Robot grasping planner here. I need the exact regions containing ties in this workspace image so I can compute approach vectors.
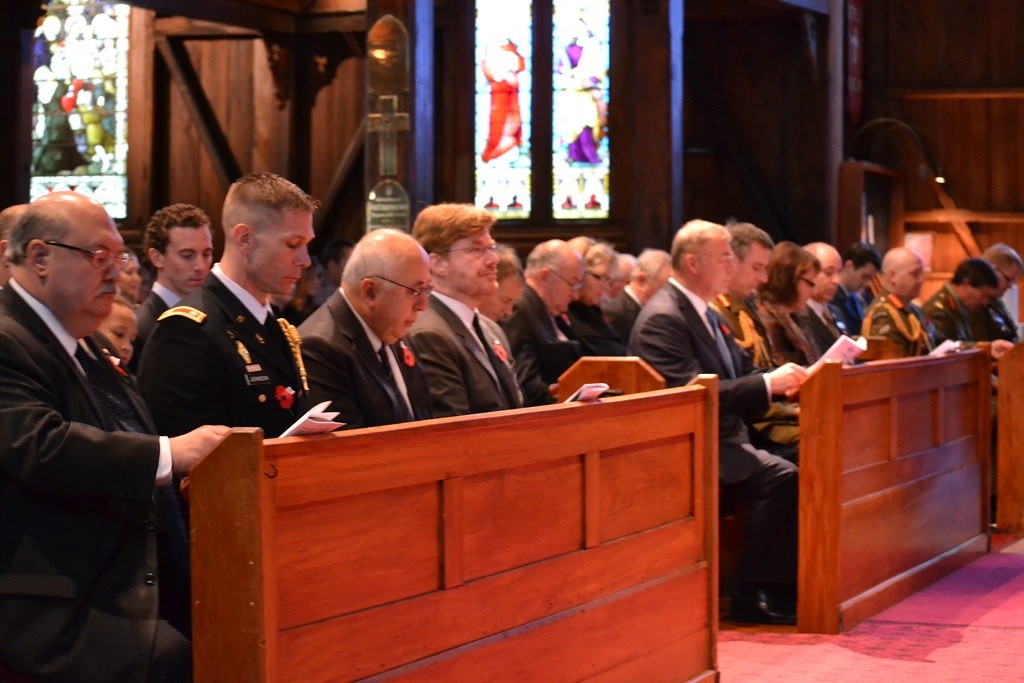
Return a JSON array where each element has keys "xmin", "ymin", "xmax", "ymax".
[
  {"xmin": 706, "ymin": 307, "xmax": 736, "ymax": 378},
  {"xmin": 378, "ymin": 342, "xmax": 413, "ymax": 422},
  {"xmin": 473, "ymin": 314, "xmax": 523, "ymax": 408},
  {"xmin": 824, "ymin": 314, "xmax": 840, "ymax": 338},
  {"xmin": 73, "ymin": 341, "xmax": 190, "ymax": 563},
  {"xmin": 264, "ymin": 311, "xmax": 300, "ymax": 377}
]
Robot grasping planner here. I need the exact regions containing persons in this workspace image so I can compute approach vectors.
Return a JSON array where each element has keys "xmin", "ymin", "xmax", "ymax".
[
  {"xmin": 802, "ymin": 242, "xmax": 864, "ymax": 366},
  {"xmin": 97, "ymin": 203, "xmax": 213, "ymax": 365},
  {"xmin": 709, "ymin": 222, "xmax": 800, "ymax": 464},
  {"xmin": 861, "ymin": 246, "xmax": 1015, "ymax": 420},
  {"xmin": 0, "ymin": 191, "xmax": 230, "ymax": 683},
  {"xmin": 755, "ymin": 241, "xmax": 821, "ymax": 365},
  {"xmin": 138, "ymin": 171, "xmax": 322, "ymax": 638},
  {"xmin": 628, "ymin": 218, "xmax": 808, "ymax": 624},
  {"xmin": 0, "ymin": 204, "xmax": 30, "ymax": 289},
  {"xmin": 827, "ymin": 241, "xmax": 885, "ymax": 334},
  {"xmin": 405, "ymin": 203, "xmax": 526, "ymax": 422},
  {"xmin": 922, "ymin": 258, "xmax": 999, "ymax": 340},
  {"xmin": 496, "ymin": 237, "xmax": 673, "ymax": 408},
  {"xmin": 272, "ymin": 236, "xmax": 357, "ymax": 327},
  {"xmin": 981, "ymin": 243, "xmax": 1024, "ymax": 337},
  {"xmin": 299, "ymin": 227, "xmax": 434, "ymax": 429}
]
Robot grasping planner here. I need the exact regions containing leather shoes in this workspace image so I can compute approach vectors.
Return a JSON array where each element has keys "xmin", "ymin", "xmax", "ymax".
[{"xmin": 729, "ymin": 589, "xmax": 796, "ymax": 623}]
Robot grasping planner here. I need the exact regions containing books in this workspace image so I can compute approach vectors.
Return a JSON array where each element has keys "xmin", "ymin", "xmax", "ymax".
[
  {"xmin": 807, "ymin": 335, "xmax": 867, "ymax": 369},
  {"xmin": 279, "ymin": 401, "xmax": 346, "ymax": 438},
  {"xmin": 564, "ymin": 383, "xmax": 609, "ymax": 401}
]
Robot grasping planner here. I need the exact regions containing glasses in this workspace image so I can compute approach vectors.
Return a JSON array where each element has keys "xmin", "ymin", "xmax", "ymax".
[
  {"xmin": 997, "ymin": 267, "xmax": 1015, "ymax": 286},
  {"xmin": 22, "ymin": 238, "xmax": 133, "ymax": 270},
  {"xmin": 361, "ymin": 275, "xmax": 435, "ymax": 300},
  {"xmin": 798, "ymin": 276, "xmax": 817, "ymax": 292},
  {"xmin": 549, "ymin": 268, "xmax": 583, "ymax": 291},
  {"xmin": 441, "ymin": 244, "xmax": 500, "ymax": 258},
  {"xmin": 591, "ymin": 272, "xmax": 605, "ymax": 283}
]
